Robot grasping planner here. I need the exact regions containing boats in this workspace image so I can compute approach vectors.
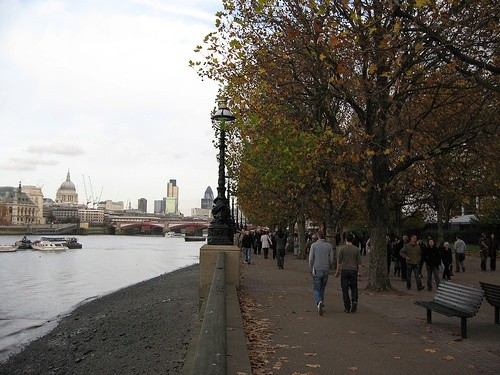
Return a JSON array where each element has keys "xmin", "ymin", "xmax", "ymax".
[
  {"xmin": 165, "ymin": 230, "xmax": 185, "ymax": 238},
  {"xmin": 0, "ymin": 244, "xmax": 19, "ymax": 252},
  {"xmin": 15, "ymin": 235, "xmax": 33, "ymax": 249},
  {"xmin": 184, "ymin": 236, "xmax": 207, "ymax": 241},
  {"xmin": 31, "ymin": 237, "xmax": 82, "ymax": 251}
]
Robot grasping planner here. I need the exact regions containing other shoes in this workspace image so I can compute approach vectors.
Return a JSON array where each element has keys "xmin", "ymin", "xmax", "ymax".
[
  {"xmin": 351, "ymin": 303, "xmax": 357, "ymax": 313},
  {"xmin": 344, "ymin": 309, "xmax": 350, "ymax": 313},
  {"xmin": 318, "ymin": 302, "xmax": 323, "ymax": 316},
  {"xmin": 418, "ymin": 286, "xmax": 425, "ymax": 291}
]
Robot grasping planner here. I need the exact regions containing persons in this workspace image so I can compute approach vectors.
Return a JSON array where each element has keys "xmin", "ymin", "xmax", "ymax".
[
  {"xmin": 309, "ymin": 229, "xmax": 333, "ymax": 315},
  {"xmin": 294, "ymin": 232, "xmax": 366, "ymax": 256},
  {"xmin": 242, "ymin": 229, "xmax": 287, "ymax": 269},
  {"xmin": 452, "ymin": 234, "xmax": 466, "ymax": 272},
  {"xmin": 480, "ymin": 234, "xmax": 496, "ymax": 272},
  {"xmin": 366, "ymin": 233, "xmax": 455, "ymax": 291},
  {"xmin": 334, "ymin": 234, "xmax": 362, "ymax": 313}
]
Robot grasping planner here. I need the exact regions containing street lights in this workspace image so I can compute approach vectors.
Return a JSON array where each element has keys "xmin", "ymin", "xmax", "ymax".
[{"xmin": 206, "ymin": 94, "xmax": 250, "ymax": 246}]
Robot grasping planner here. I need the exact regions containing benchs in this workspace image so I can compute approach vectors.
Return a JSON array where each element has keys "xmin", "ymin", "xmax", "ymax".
[
  {"xmin": 479, "ymin": 280, "xmax": 500, "ymax": 324},
  {"xmin": 413, "ymin": 281, "xmax": 484, "ymax": 339}
]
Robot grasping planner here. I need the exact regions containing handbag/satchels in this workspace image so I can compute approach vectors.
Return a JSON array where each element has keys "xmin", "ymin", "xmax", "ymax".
[
  {"xmin": 477, "ymin": 251, "xmax": 481, "ymax": 257},
  {"xmin": 459, "ymin": 253, "xmax": 465, "ymax": 261}
]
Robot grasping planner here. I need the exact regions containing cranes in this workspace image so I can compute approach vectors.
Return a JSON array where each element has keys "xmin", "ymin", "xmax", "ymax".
[{"xmin": 82, "ymin": 174, "xmax": 104, "ymax": 202}]
